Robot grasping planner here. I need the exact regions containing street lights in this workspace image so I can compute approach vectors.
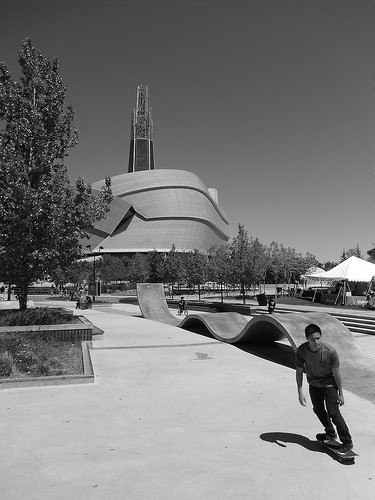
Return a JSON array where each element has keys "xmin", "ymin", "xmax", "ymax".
[{"xmin": 86, "ymin": 245, "xmax": 104, "ymax": 301}]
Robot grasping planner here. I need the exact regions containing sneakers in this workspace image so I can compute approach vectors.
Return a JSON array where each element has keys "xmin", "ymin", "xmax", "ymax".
[
  {"xmin": 337, "ymin": 443, "xmax": 352, "ymax": 455},
  {"xmin": 319, "ymin": 433, "xmax": 337, "ymax": 441}
]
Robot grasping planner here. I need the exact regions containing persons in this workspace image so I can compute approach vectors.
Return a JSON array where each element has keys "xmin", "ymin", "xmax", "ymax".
[
  {"xmin": 179, "ymin": 297, "xmax": 186, "ymax": 312},
  {"xmin": 81, "ymin": 296, "xmax": 91, "ymax": 310},
  {"xmin": 268, "ymin": 296, "xmax": 276, "ymax": 314},
  {"xmin": 296, "ymin": 324, "xmax": 354, "ymax": 456}
]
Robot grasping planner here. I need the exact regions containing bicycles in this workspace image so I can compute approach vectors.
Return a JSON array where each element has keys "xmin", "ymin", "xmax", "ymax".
[{"xmin": 177, "ymin": 304, "xmax": 188, "ymax": 317}]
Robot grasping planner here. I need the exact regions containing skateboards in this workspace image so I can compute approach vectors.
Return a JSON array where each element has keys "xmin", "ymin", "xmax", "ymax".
[{"xmin": 316, "ymin": 433, "xmax": 360, "ymax": 463}]
